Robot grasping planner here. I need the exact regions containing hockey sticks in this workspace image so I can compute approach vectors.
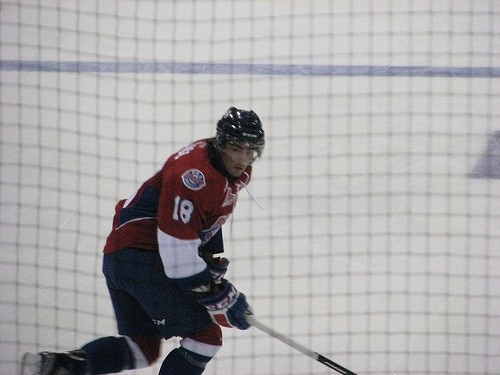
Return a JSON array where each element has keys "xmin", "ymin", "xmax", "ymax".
[{"xmin": 244, "ymin": 313, "xmax": 360, "ymax": 375}]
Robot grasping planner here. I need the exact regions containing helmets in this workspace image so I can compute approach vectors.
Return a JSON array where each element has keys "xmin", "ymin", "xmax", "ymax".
[{"xmin": 214, "ymin": 107, "xmax": 264, "ymax": 161}]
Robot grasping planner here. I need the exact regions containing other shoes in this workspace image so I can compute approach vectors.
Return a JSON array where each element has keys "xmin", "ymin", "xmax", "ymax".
[{"xmin": 18, "ymin": 346, "xmax": 56, "ymax": 375}]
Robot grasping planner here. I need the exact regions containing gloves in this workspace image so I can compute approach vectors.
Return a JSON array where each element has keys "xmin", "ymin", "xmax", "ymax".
[{"xmin": 201, "ymin": 284, "xmax": 252, "ymax": 333}]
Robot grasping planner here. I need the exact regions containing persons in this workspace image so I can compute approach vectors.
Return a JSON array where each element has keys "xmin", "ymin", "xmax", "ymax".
[{"xmin": 22, "ymin": 108, "xmax": 265, "ymax": 375}]
